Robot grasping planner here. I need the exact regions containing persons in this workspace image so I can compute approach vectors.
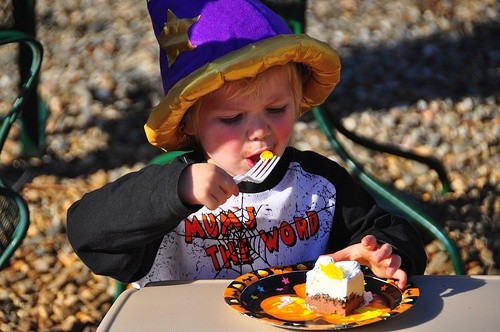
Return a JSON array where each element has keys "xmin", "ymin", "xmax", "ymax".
[{"xmin": 66, "ymin": 0, "xmax": 428, "ymax": 290}]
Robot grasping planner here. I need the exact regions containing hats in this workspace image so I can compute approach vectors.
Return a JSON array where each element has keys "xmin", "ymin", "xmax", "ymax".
[{"xmin": 143, "ymin": 0, "xmax": 341, "ymax": 153}]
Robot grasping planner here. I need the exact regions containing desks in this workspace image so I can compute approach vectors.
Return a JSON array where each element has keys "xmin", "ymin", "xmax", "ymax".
[{"xmin": 95, "ymin": 273, "xmax": 500, "ymax": 332}]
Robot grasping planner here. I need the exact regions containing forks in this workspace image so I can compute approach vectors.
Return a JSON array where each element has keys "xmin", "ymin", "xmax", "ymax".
[{"xmin": 233, "ymin": 154, "xmax": 281, "ymax": 183}]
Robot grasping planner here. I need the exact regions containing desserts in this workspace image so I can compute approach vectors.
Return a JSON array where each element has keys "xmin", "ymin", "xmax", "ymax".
[{"xmin": 304, "ymin": 256, "xmax": 367, "ymax": 315}]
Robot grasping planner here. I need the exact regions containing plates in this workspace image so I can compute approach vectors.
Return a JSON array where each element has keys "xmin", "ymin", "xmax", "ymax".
[{"xmin": 224, "ymin": 263, "xmax": 420, "ymax": 330}]
